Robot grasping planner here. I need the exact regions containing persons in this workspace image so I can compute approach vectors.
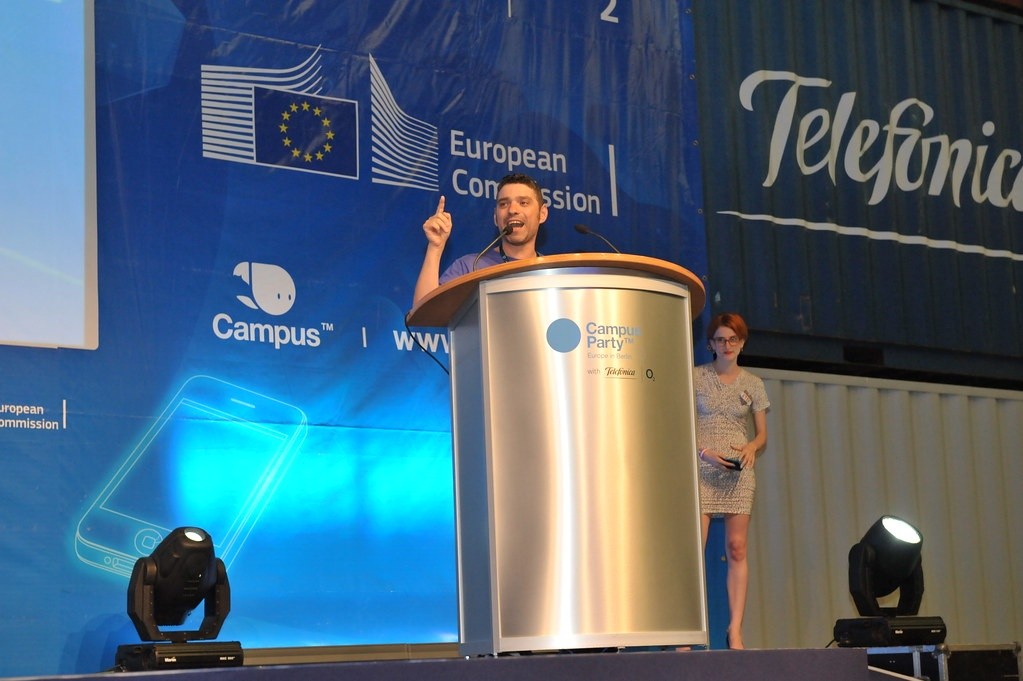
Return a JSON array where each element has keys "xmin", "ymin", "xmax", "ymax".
[
  {"xmin": 413, "ymin": 170, "xmax": 555, "ymax": 306},
  {"xmin": 675, "ymin": 314, "xmax": 772, "ymax": 653}
]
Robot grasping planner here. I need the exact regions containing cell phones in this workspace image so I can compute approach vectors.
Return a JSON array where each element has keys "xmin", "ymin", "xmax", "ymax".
[{"xmin": 723, "ymin": 459, "xmax": 742, "ymax": 471}]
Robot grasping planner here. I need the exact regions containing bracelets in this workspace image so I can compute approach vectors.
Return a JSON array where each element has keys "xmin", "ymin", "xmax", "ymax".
[{"xmin": 700, "ymin": 448, "xmax": 707, "ymax": 459}]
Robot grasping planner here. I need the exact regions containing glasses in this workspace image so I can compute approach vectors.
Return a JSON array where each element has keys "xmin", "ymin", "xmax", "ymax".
[{"xmin": 710, "ymin": 336, "xmax": 740, "ymax": 347}]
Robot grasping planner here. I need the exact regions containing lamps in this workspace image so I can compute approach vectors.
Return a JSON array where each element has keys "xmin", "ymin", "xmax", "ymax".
[
  {"xmin": 115, "ymin": 525, "xmax": 243, "ymax": 672},
  {"xmin": 829, "ymin": 516, "xmax": 950, "ymax": 652}
]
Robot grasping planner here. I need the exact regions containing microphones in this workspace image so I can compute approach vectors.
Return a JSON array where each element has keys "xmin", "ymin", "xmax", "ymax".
[
  {"xmin": 473, "ymin": 224, "xmax": 513, "ymax": 271},
  {"xmin": 574, "ymin": 223, "xmax": 622, "ymax": 253}
]
